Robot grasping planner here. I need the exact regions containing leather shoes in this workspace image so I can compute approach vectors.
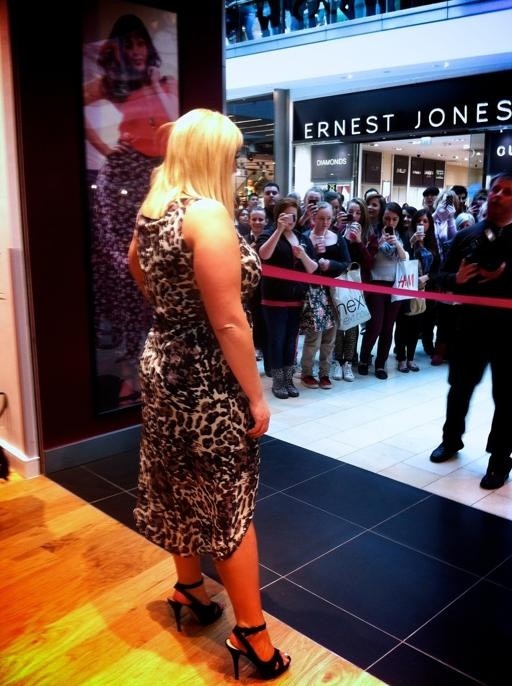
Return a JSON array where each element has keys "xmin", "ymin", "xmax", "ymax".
[
  {"xmin": 407, "ymin": 361, "xmax": 419, "ymax": 371},
  {"xmin": 398, "ymin": 364, "xmax": 410, "ymax": 373}
]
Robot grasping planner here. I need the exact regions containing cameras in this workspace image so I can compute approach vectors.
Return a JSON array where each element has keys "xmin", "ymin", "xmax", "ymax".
[
  {"xmin": 340, "ymin": 212, "xmax": 353, "ymax": 224},
  {"xmin": 284, "ymin": 214, "xmax": 296, "ymax": 224},
  {"xmin": 417, "ymin": 225, "xmax": 425, "ymax": 234},
  {"xmin": 464, "ymin": 225, "xmax": 503, "ymax": 271},
  {"xmin": 385, "ymin": 226, "xmax": 394, "ymax": 236},
  {"xmin": 308, "ymin": 200, "xmax": 316, "ymax": 210}
]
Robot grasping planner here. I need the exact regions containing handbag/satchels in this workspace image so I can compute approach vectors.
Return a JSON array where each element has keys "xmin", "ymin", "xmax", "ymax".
[
  {"xmin": 329, "ymin": 264, "xmax": 375, "ymax": 330},
  {"xmin": 391, "ymin": 251, "xmax": 419, "ymax": 301},
  {"xmin": 404, "ymin": 287, "xmax": 429, "ymax": 315},
  {"xmin": 299, "ymin": 286, "xmax": 337, "ymax": 333}
]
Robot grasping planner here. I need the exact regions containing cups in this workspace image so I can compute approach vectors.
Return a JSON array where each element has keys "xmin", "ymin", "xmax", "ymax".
[
  {"xmin": 345, "ymin": 221, "xmax": 359, "ymax": 241},
  {"xmin": 314, "ymin": 235, "xmax": 325, "ymax": 254}
]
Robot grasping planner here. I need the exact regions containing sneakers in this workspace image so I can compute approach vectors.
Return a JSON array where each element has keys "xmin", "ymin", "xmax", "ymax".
[
  {"xmin": 285, "ymin": 381, "xmax": 299, "ymax": 397},
  {"xmin": 331, "ymin": 364, "xmax": 342, "ymax": 380},
  {"xmin": 343, "ymin": 363, "xmax": 354, "ymax": 382},
  {"xmin": 376, "ymin": 369, "xmax": 389, "ymax": 380},
  {"xmin": 358, "ymin": 363, "xmax": 369, "ymax": 376},
  {"xmin": 479, "ymin": 458, "xmax": 511, "ymax": 489},
  {"xmin": 431, "ymin": 440, "xmax": 465, "ymax": 463},
  {"xmin": 318, "ymin": 376, "xmax": 331, "ymax": 388},
  {"xmin": 301, "ymin": 375, "xmax": 320, "ymax": 389},
  {"xmin": 272, "ymin": 383, "xmax": 289, "ymax": 399}
]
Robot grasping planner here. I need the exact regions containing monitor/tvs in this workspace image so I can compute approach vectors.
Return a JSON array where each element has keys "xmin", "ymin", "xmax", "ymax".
[{"xmin": 311, "ymin": 144, "xmax": 353, "ymax": 180}]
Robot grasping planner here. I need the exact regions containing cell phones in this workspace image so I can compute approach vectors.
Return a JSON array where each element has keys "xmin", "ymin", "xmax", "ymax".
[{"xmin": 446, "ymin": 195, "xmax": 453, "ymax": 208}]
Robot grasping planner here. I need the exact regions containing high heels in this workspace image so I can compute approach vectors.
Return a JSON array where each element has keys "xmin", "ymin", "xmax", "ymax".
[
  {"xmin": 169, "ymin": 579, "xmax": 224, "ymax": 632},
  {"xmin": 225, "ymin": 622, "xmax": 292, "ymax": 680}
]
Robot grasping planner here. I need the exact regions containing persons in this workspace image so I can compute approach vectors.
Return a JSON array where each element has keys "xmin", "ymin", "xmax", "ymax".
[
  {"xmin": 225, "ymin": 0, "xmax": 400, "ymax": 46},
  {"xmin": 123, "ymin": 104, "xmax": 298, "ymax": 683},
  {"xmin": 79, "ymin": 11, "xmax": 180, "ymax": 406},
  {"xmin": 427, "ymin": 166, "xmax": 511, "ymax": 490}
]
